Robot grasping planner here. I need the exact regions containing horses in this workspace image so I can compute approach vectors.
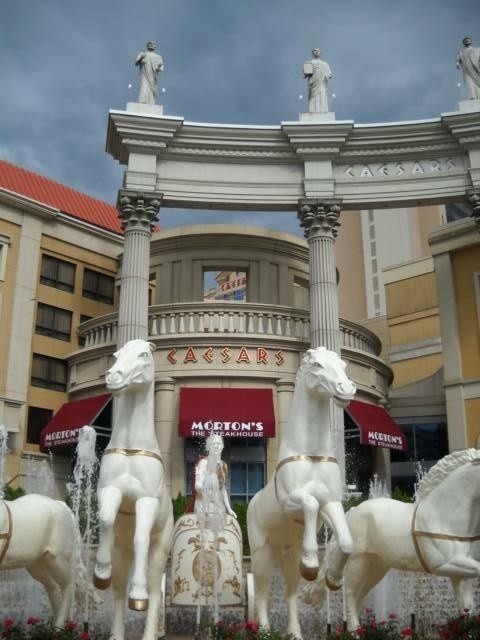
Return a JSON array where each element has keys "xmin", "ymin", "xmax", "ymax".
[
  {"xmin": 244, "ymin": 346, "xmax": 359, "ymax": 640},
  {"xmin": 0, "ymin": 491, "xmax": 104, "ymax": 638},
  {"xmin": 296, "ymin": 445, "xmax": 480, "ymax": 640},
  {"xmin": 87, "ymin": 338, "xmax": 177, "ymax": 640}
]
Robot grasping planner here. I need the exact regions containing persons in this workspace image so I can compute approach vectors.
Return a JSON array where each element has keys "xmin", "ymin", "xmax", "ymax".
[
  {"xmin": 192, "ymin": 433, "xmax": 239, "ymax": 521},
  {"xmin": 302, "ymin": 48, "xmax": 334, "ymax": 113},
  {"xmin": 455, "ymin": 34, "xmax": 480, "ymax": 101},
  {"xmin": 134, "ymin": 40, "xmax": 165, "ymax": 104}
]
